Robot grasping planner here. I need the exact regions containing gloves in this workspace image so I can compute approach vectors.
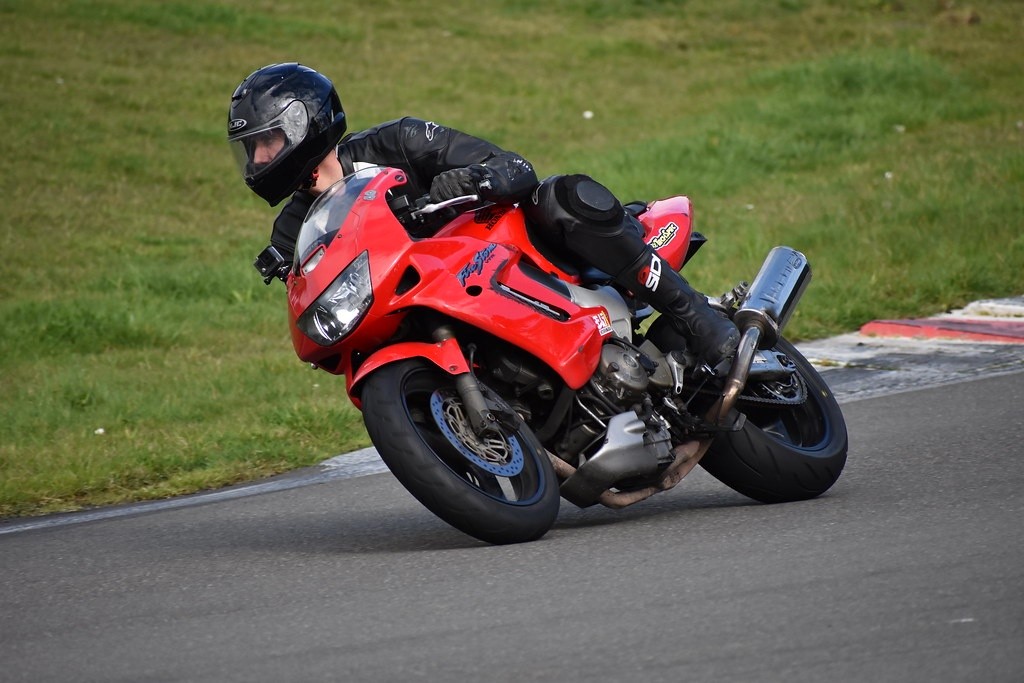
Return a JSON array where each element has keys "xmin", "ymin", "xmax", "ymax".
[{"xmin": 430, "ymin": 165, "xmax": 490, "ymax": 214}]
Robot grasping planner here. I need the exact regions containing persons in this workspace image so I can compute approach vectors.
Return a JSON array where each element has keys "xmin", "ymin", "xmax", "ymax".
[{"xmin": 225, "ymin": 61, "xmax": 742, "ymax": 366}]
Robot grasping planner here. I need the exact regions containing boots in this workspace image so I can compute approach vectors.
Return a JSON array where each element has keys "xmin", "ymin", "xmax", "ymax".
[{"xmin": 616, "ymin": 245, "xmax": 741, "ymax": 367}]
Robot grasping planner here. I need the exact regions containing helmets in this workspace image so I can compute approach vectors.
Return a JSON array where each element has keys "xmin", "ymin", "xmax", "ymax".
[{"xmin": 225, "ymin": 61, "xmax": 348, "ymax": 207}]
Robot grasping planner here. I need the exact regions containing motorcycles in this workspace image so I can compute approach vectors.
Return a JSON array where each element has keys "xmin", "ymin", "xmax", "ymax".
[{"xmin": 252, "ymin": 161, "xmax": 849, "ymax": 545}]
